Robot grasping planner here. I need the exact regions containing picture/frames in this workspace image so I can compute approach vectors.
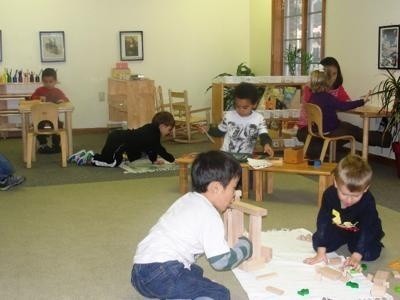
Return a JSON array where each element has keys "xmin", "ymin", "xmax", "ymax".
[
  {"xmin": 38, "ymin": 30, "xmax": 66, "ymax": 62},
  {"xmin": 119, "ymin": 30, "xmax": 144, "ymax": 63},
  {"xmin": 377, "ymin": 25, "xmax": 400, "ymax": 70},
  {"xmin": 0, "ymin": 29, "xmax": 4, "ymax": 62}
]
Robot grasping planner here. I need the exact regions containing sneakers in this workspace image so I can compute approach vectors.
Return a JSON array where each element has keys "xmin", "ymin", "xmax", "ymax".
[
  {"xmin": 66, "ymin": 149, "xmax": 86, "ymax": 162},
  {"xmin": 0, "ymin": 174, "xmax": 25, "ymax": 191},
  {"xmin": 77, "ymin": 150, "xmax": 96, "ymax": 166},
  {"xmin": 37, "ymin": 145, "xmax": 61, "ymax": 155}
]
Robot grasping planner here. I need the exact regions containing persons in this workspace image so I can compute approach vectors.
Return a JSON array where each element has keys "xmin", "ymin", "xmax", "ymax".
[
  {"xmin": 125, "ymin": 36, "xmax": 138, "ymax": 57},
  {"xmin": 297, "ymin": 56, "xmax": 351, "ymax": 159},
  {"xmin": 0, "ymin": 153, "xmax": 26, "ymax": 192},
  {"xmin": 308, "ymin": 69, "xmax": 391, "ymax": 159},
  {"xmin": 131, "ymin": 150, "xmax": 258, "ymax": 300},
  {"xmin": 31, "ymin": 68, "xmax": 69, "ymax": 153},
  {"xmin": 303, "ymin": 154, "xmax": 386, "ymax": 270},
  {"xmin": 196, "ymin": 82, "xmax": 275, "ymax": 192},
  {"xmin": 67, "ymin": 111, "xmax": 178, "ymax": 167}
]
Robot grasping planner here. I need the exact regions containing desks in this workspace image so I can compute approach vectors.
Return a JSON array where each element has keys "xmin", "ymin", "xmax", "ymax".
[
  {"xmin": 344, "ymin": 104, "xmax": 393, "ymax": 163},
  {"xmin": 20, "ymin": 99, "xmax": 76, "ymax": 163}
]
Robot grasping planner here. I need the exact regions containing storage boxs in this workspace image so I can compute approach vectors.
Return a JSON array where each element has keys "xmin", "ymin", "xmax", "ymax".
[{"xmin": 111, "ymin": 66, "xmax": 132, "ymax": 79}]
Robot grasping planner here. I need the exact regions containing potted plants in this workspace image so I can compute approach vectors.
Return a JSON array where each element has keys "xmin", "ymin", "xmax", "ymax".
[{"xmin": 366, "ymin": 62, "xmax": 399, "ymax": 172}]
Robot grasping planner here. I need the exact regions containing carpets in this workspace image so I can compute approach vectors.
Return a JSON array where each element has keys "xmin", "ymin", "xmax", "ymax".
[{"xmin": 0, "ymin": 169, "xmax": 399, "ymax": 300}]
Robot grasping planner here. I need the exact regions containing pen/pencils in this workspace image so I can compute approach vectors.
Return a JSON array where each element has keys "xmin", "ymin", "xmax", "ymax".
[{"xmin": 6, "ymin": 67, "xmax": 43, "ymax": 77}]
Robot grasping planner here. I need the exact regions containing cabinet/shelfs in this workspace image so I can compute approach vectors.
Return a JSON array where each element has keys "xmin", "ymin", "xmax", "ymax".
[
  {"xmin": 212, "ymin": 76, "xmax": 312, "ymax": 155},
  {"xmin": 0, "ymin": 80, "xmax": 61, "ymax": 140},
  {"xmin": 108, "ymin": 78, "xmax": 157, "ymax": 127}
]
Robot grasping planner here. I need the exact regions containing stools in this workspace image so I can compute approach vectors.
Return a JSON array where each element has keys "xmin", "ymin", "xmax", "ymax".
[
  {"xmin": 174, "ymin": 150, "xmax": 261, "ymax": 204},
  {"xmin": 247, "ymin": 156, "xmax": 340, "ymax": 206}
]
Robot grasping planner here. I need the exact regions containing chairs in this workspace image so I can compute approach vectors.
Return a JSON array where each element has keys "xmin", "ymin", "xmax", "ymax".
[
  {"xmin": 154, "ymin": 86, "xmax": 192, "ymax": 135},
  {"xmin": 303, "ymin": 102, "xmax": 356, "ymax": 164},
  {"xmin": 168, "ymin": 88, "xmax": 212, "ymax": 144},
  {"xmin": 24, "ymin": 102, "xmax": 69, "ymax": 168}
]
